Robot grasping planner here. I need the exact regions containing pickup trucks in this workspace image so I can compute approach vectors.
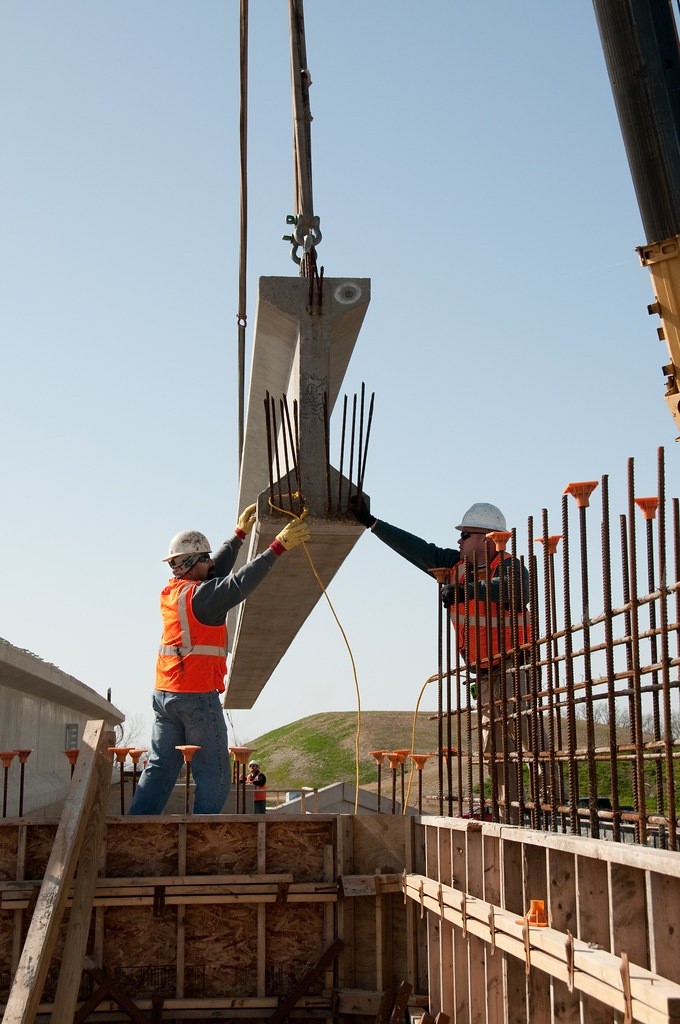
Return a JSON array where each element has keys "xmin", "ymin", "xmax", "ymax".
[{"xmin": 576, "ymin": 798, "xmax": 634, "ymax": 813}]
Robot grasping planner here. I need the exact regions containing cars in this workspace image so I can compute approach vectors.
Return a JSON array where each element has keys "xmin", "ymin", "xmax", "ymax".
[{"xmin": 456, "ymin": 806, "xmax": 493, "ymax": 821}]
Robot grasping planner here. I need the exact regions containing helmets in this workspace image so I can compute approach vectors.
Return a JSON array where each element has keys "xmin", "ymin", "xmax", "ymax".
[
  {"xmin": 248, "ymin": 760, "xmax": 259, "ymax": 770},
  {"xmin": 162, "ymin": 529, "xmax": 212, "ymax": 566},
  {"xmin": 455, "ymin": 502, "xmax": 510, "ymax": 533}
]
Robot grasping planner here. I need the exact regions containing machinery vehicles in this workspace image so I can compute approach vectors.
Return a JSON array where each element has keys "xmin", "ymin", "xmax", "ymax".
[{"xmin": 590, "ymin": 1, "xmax": 680, "ymax": 430}]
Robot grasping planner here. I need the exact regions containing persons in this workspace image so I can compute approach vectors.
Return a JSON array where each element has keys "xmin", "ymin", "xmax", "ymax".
[
  {"xmin": 347, "ymin": 496, "xmax": 570, "ymax": 824},
  {"xmin": 128, "ymin": 502, "xmax": 312, "ymax": 814},
  {"xmin": 240, "ymin": 760, "xmax": 266, "ymax": 814}
]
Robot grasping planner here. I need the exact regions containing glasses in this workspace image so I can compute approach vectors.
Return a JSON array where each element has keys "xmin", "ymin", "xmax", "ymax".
[
  {"xmin": 197, "ymin": 554, "xmax": 210, "ymax": 562},
  {"xmin": 461, "ymin": 530, "xmax": 487, "ymax": 542}
]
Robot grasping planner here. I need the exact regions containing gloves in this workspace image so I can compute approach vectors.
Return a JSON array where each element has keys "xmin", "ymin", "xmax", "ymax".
[
  {"xmin": 270, "ymin": 518, "xmax": 311, "ymax": 556},
  {"xmin": 441, "ymin": 583, "xmax": 475, "ymax": 607},
  {"xmin": 232, "ymin": 504, "xmax": 259, "ymax": 539}
]
